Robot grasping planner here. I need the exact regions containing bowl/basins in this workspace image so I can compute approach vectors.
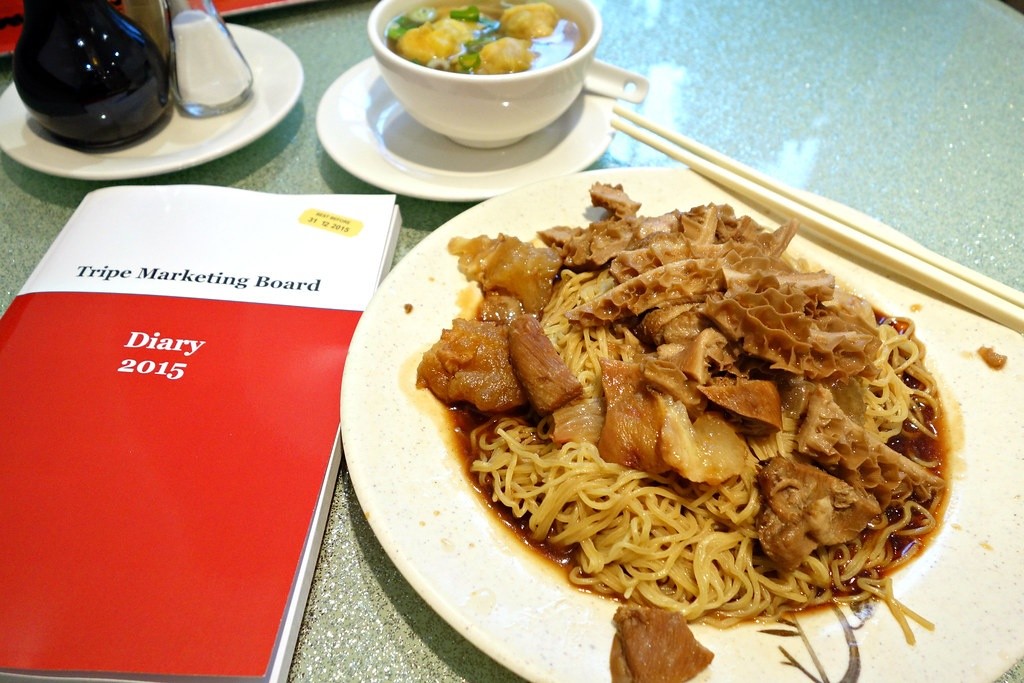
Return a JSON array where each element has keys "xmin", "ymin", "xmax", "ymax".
[{"xmin": 367, "ymin": 0, "xmax": 603, "ymax": 148}]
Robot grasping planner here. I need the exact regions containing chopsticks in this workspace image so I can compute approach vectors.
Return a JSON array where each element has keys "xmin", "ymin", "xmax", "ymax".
[{"xmin": 610, "ymin": 106, "xmax": 1024, "ymax": 336}]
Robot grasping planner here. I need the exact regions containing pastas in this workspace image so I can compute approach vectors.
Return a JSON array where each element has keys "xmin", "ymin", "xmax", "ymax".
[{"xmin": 464, "ymin": 265, "xmax": 943, "ymax": 648}]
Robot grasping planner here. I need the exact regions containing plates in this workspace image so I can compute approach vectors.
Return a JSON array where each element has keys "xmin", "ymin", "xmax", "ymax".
[
  {"xmin": 316, "ymin": 55, "xmax": 616, "ymax": 202},
  {"xmin": 0, "ymin": 23, "xmax": 304, "ymax": 180},
  {"xmin": 341, "ymin": 169, "xmax": 1023, "ymax": 683}
]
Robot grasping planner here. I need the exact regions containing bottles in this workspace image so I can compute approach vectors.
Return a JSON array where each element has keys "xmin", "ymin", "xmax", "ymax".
[
  {"xmin": 165, "ymin": 0, "xmax": 254, "ymax": 120},
  {"xmin": 12, "ymin": 1, "xmax": 168, "ymax": 151}
]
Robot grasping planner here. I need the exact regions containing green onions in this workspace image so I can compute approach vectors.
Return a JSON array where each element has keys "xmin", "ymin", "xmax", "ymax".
[{"xmin": 385, "ymin": 6, "xmax": 504, "ymax": 74}]
